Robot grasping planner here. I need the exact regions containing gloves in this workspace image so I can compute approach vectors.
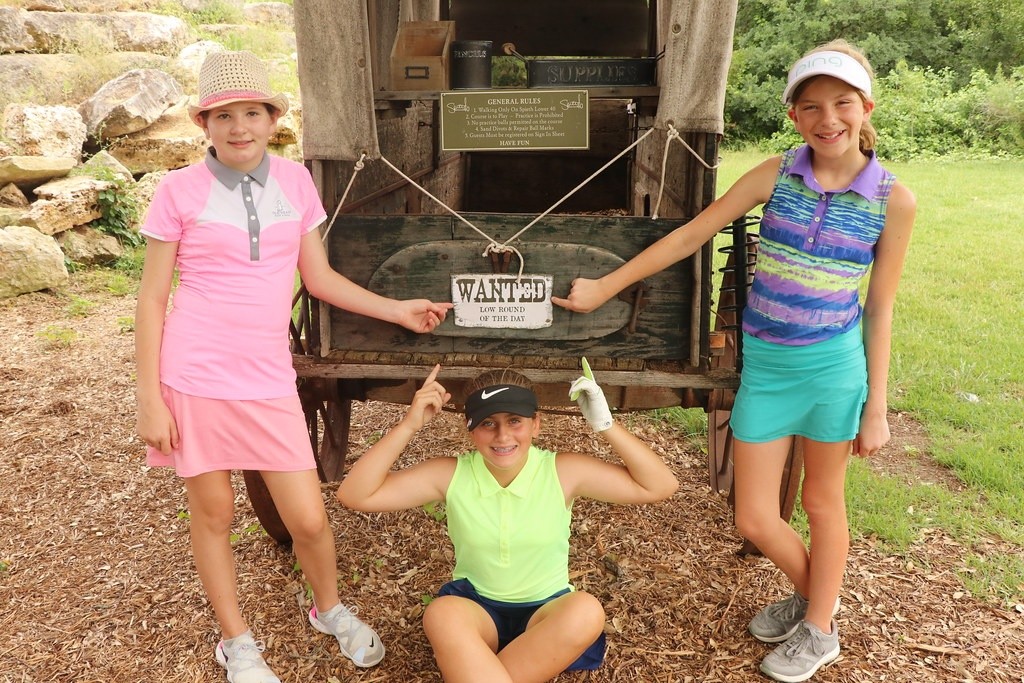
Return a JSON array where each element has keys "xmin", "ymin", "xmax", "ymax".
[{"xmin": 566, "ymin": 355, "xmax": 614, "ymax": 432}]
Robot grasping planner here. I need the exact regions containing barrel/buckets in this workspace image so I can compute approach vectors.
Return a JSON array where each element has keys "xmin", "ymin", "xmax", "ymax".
[{"xmin": 450, "ymin": 39, "xmax": 493, "ymax": 89}]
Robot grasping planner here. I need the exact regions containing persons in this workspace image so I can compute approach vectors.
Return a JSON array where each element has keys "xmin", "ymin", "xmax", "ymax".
[
  {"xmin": 338, "ymin": 355, "xmax": 680, "ymax": 683},
  {"xmin": 135, "ymin": 51, "xmax": 456, "ymax": 683},
  {"xmin": 550, "ymin": 38, "xmax": 917, "ymax": 683}
]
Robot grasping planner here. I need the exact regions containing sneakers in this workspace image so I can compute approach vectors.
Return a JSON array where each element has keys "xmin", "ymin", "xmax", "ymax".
[
  {"xmin": 216, "ymin": 637, "xmax": 282, "ymax": 683},
  {"xmin": 748, "ymin": 590, "xmax": 842, "ymax": 642},
  {"xmin": 758, "ymin": 616, "xmax": 841, "ymax": 683},
  {"xmin": 308, "ymin": 598, "xmax": 385, "ymax": 668}
]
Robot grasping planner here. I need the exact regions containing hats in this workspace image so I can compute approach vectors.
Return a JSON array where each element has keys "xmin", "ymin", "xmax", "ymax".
[
  {"xmin": 782, "ymin": 50, "xmax": 872, "ymax": 105},
  {"xmin": 187, "ymin": 50, "xmax": 289, "ymax": 125},
  {"xmin": 464, "ymin": 385, "xmax": 538, "ymax": 432}
]
